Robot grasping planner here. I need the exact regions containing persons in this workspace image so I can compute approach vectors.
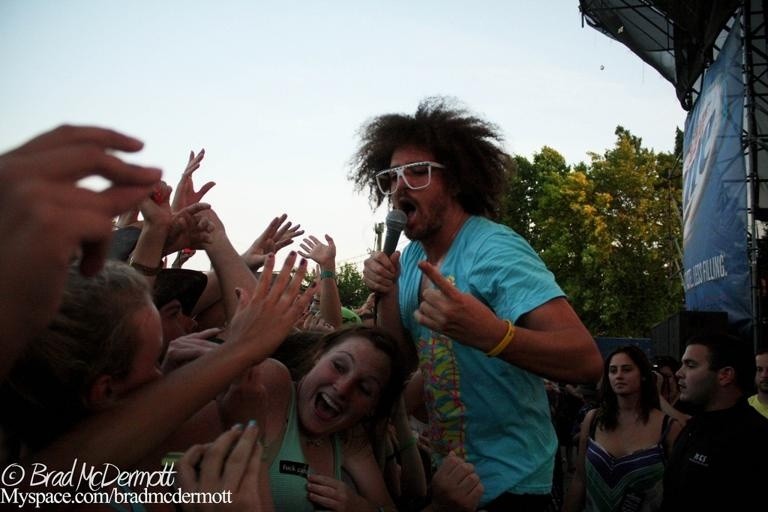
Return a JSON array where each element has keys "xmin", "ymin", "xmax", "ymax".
[
  {"xmin": 346, "ymin": 92, "xmax": 606, "ymax": 512},
  {"xmin": 2, "ymin": 121, "xmax": 768, "ymax": 509}
]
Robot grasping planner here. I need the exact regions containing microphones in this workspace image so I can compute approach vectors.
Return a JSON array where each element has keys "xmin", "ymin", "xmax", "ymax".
[{"xmin": 373, "ymin": 209, "xmax": 408, "ymax": 303}]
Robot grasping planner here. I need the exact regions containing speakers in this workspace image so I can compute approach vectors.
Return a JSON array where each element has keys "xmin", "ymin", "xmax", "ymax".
[{"xmin": 651, "ymin": 311, "xmax": 729, "ymax": 365}]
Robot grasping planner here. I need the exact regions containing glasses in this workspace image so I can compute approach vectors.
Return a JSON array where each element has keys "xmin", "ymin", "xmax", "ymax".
[{"xmin": 375, "ymin": 161, "xmax": 445, "ymax": 195}]
